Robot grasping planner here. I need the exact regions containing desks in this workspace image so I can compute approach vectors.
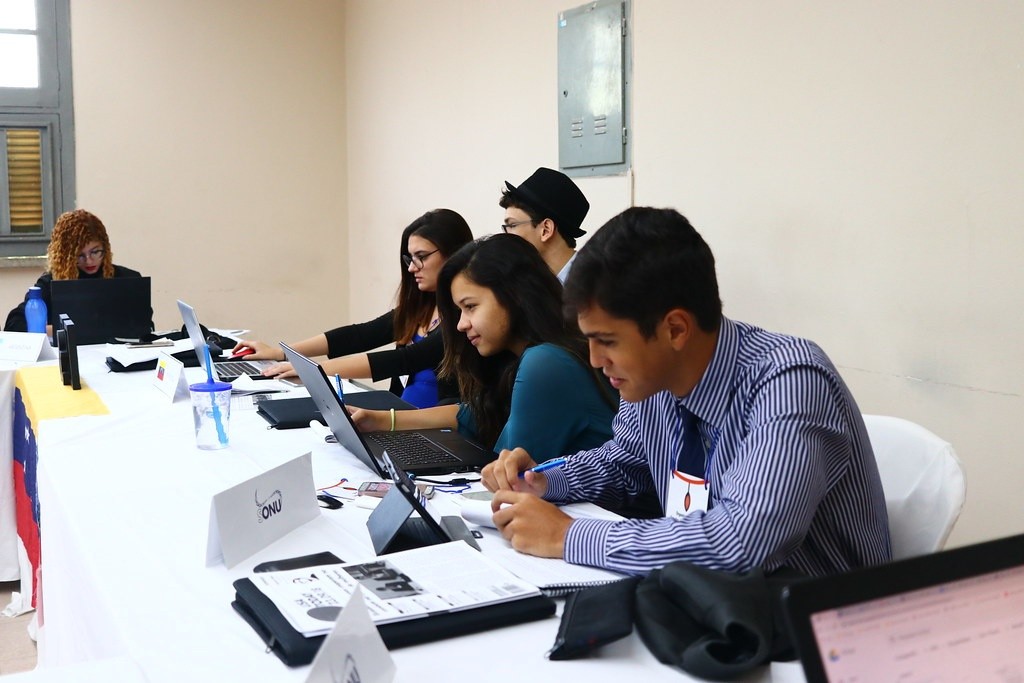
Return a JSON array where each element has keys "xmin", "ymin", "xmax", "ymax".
[{"xmin": 0, "ymin": 318, "xmax": 791, "ymax": 682}]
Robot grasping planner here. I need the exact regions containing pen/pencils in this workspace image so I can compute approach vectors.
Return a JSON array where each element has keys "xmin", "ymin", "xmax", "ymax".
[
  {"xmin": 518, "ymin": 458, "xmax": 567, "ymax": 478},
  {"xmin": 334, "ymin": 373, "xmax": 345, "ymax": 405}
]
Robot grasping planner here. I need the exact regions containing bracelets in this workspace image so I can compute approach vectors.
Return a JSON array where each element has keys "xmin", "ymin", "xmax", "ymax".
[
  {"xmin": 284, "ymin": 354, "xmax": 287, "ymax": 362},
  {"xmin": 389, "ymin": 409, "xmax": 395, "ymax": 433}
]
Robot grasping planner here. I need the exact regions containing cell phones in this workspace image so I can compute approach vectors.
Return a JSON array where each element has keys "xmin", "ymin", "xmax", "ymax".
[
  {"xmin": 356, "ymin": 482, "xmax": 435, "ymax": 500},
  {"xmin": 281, "ymin": 376, "xmax": 303, "ymax": 387}
]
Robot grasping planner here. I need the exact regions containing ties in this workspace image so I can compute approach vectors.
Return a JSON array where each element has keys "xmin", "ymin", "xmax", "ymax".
[{"xmin": 678, "ymin": 406, "xmax": 705, "ymax": 478}]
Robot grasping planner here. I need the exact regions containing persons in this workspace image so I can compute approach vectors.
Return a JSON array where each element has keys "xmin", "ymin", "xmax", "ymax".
[
  {"xmin": 343, "ymin": 232, "xmax": 661, "ymax": 522},
  {"xmin": 5, "ymin": 211, "xmax": 155, "ymax": 343},
  {"xmin": 501, "ymin": 167, "xmax": 590, "ymax": 286},
  {"xmin": 232, "ymin": 209, "xmax": 473, "ymax": 400},
  {"xmin": 481, "ymin": 207, "xmax": 893, "ymax": 586}
]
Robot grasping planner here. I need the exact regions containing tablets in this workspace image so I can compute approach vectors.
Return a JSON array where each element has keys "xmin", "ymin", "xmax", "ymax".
[{"xmin": 382, "ymin": 449, "xmax": 453, "ymax": 542}]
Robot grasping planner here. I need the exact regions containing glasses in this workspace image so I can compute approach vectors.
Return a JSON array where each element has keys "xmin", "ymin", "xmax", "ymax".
[
  {"xmin": 502, "ymin": 220, "xmax": 532, "ymax": 234},
  {"xmin": 78, "ymin": 249, "xmax": 104, "ymax": 262},
  {"xmin": 403, "ymin": 249, "xmax": 439, "ymax": 269}
]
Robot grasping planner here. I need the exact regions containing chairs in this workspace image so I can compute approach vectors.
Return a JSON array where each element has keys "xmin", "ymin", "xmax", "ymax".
[{"xmin": 859, "ymin": 413, "xmax": 966, "ymax": 559}]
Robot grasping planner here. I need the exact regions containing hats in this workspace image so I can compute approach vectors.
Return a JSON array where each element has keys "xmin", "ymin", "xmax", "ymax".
[{"xmin": 505, "ymin": 167, "xmax": 589, "ymax": 238}]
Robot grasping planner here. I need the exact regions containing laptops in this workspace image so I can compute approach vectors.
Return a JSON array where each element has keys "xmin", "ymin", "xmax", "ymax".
[
  {"xmin": 278, "ymin": 342, "xmax": 499, "ymax": 479},
  {"xmin": 50, "ymin": 276, "xmax": 152, "ymax": 347},
  {"xmin": 176, "ymin": 298, "xmax": 284, "ymax": 382}
]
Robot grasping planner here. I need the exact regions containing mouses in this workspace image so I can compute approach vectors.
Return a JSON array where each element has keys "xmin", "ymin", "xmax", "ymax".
[{"xmin": 227, "ymin": 348, "xmax": 255, "ymax": 362}]
